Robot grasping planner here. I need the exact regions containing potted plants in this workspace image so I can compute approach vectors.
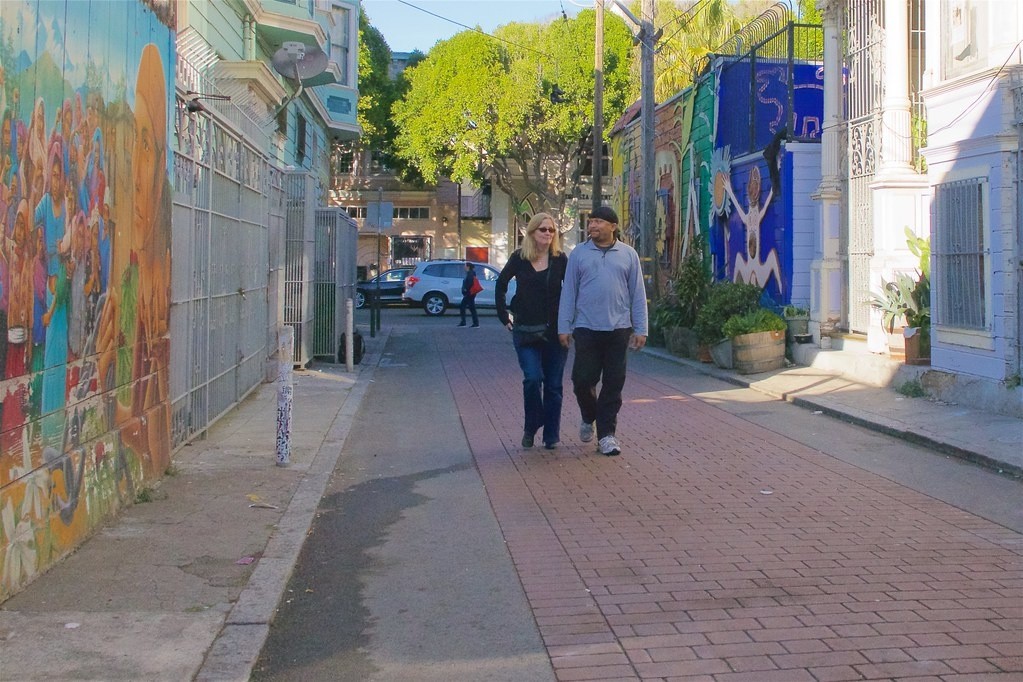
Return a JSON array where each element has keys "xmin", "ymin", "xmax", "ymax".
[
  {"xmin": 644, "ymin": 232, "xmax": 811, "ymax": 376},
  {"xmin": 866, "ymin": 226, "xmax": 932, "ymax": 366}
]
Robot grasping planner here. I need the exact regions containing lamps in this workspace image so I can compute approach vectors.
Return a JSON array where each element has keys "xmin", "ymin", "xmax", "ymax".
[{"xmin": 443, "ymin": 217, "xmax": 448, "ymax": 223}]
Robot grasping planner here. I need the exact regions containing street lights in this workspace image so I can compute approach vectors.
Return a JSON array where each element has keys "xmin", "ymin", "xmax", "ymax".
[{"xmin": 376, "ymin": 186, "xmax": 384, "ymax": 335}]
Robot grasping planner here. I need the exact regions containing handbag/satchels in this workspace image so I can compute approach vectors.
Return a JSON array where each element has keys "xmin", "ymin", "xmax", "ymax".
[
  {"xmin": 468, "ymin": 275, "xmax": 483, "ymax": 296},
  {"xmin": 517, "ymin": 324, "xmax": 552, "ymax": 347}
]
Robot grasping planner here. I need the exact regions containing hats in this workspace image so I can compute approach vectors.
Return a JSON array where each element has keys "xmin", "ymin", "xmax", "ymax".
[{"xmin": 588, "ymin": 207, "xmax": 619, "ymax": 224}]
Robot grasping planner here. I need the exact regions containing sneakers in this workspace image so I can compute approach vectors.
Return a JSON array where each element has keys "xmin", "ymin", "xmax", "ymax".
[
  {"xmin": 598, "ymin": 435, "xmax": 621, "ymax": 455},
  {"xmin": 578, "ymin": 421, "xmax": 594, "ymax": 442}
]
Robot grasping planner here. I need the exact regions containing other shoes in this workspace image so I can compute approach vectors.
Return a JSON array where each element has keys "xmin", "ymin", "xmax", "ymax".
[
  {"xmin": 546, "ymin": 442, "xmax": 557, "ymax": 449},
  {"xmin": 522, "ymin": 433, "xmax": 533, "ymax": 447},
  {"xmin": 470, "ymin": 324, "xmax": 480, "ymax": 328},
  {"xmin": 456, "ymin": 323, "xmax": 466, "ymax": 327}
]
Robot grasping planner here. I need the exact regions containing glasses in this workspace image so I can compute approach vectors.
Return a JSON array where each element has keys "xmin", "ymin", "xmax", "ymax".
[{"xmin": 537, "ymin": 227, "xmax": 555, "ymax": 233}]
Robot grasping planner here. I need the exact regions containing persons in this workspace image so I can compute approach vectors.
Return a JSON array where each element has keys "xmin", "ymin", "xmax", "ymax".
[
  {"xmin": 457, "ymin": 263, "xmax": 480, "ymax": 328},
  {"xmin": 558, "ymin": 207, "xmax": 649, "ymax": 454},
  {"xmin": 495, "ymin": 212, "xmax": 568, "ymax": 449}
]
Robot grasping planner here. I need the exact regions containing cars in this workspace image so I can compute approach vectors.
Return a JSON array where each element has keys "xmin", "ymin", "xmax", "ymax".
[
  {"xmin": 355, "ymin": 269, "xmax": 413, "ymax": 307},
  {"xmin": 402, "ymin": 259, "xmax": 516, "ymax": 315}
]
